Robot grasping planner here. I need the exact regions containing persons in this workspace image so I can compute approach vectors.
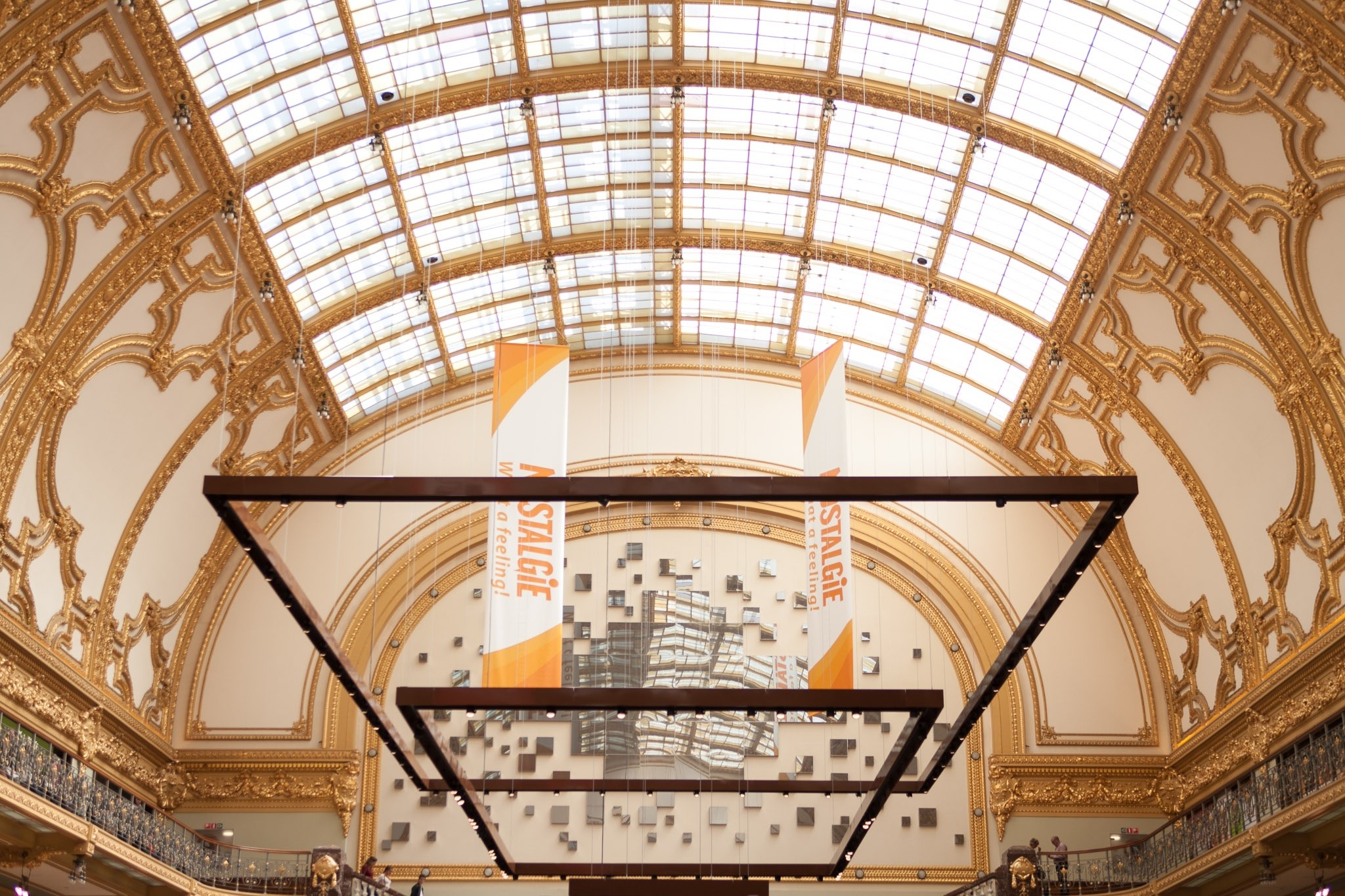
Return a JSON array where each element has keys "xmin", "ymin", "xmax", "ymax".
[
  {"xmin": 1029, "ymin": 838, "xmax": 1041, "ymax": 859},
  {"xmin": 373, "ymin": 866, "xmax": 393, "ymax": 896},
  {"xmin": 1046, "ymin": 836, "xmax": 1070, "ymax": 896},
  {"xmin": 410, "ymin": 874, "xmax": 426, "ymax": 896},
  {"xmin": 361, "ymin": 856, "xmax": 377, "ymax": 896}
]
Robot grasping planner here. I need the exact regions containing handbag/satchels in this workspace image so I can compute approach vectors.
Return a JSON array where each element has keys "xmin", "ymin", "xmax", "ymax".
[{"xmin": 379, "ymin": 875, "xmax": 385, "ymax": 886}]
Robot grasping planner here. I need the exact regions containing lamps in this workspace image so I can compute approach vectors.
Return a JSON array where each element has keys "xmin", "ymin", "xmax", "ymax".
[
  {"xmin": 258, "ymin": 269, "xmax": 275, "ymax": 303},
  {"xmin": 823, "ymin": 87, "xmax": 837, "ymax": 121},
  {"xmin": 509, "ymin": 790, "xmax": 912, "ymax": 798},
  {"xmin": 670, "ymin": 74, "xmax": 685, "ymax": 109},
  {"xmin": 925, "ymin": 281, "xmax": 936, "ymax": 305},
  {"xmin": 221, "ymin": 188, "xmax": 238, "ymax": 223},
  {"xmin": 1162, "ymin": 91, "xmax": 1183, "ymax": 132},
  {"xmin": 1047, "ymin": 339, "xmax": 1063, "ymax": 370},
  {"xmin": 291, "ymin": 340, "xmax": 306, "ymax": 368},
  {"xmin": 1257, "ymin": 856, "xmax": 1329, "ymax": 896},
  {"xmin": 1078, "ymin": 270, "xmax": 1096, "ymax": 304},
  {"xmin": 1220, "ymin": 0, "xmax": 1242, "ymax": 16},
  {"xmin": 799, "ymin": 249, "xmax": 812, "ymax": 279},
  {"xmin": 369, "ymin": 121, "xmax": 386, "ymax": 156},
  {"xmin": 1117, "ymin": 189, "xmax": 1135, "ymax": 225},
  {"xmin": 14, "ymin": 854, "xmax": 87, "ymax": 896},
  {"xmin": 543, "ymin": 248, "xmax": 556, "ymax": 274},
  {"xmin": 518, "ymin": 85, "xmax": 534, "ymax": 121},
  {"xmin": 172, "ymin": 89, "xmax": 193, "ymax": 131},
  {"xmin": 416, "ymin": 283, "xmax": 429, "ymax": 311},
  {"xmin": 969, "ymin": 123, "xmax": 987, "ymax": 157},
  {"xmin": 113, "ymin": 0, "xmax": 136, "ymax": 13},
  {"xmin": 467, "ymin": 710, "xmax": 862, "ymax": 720},
  {"xmin": 1018, "ymin": 399, "xmax": 1033, "ymax": 427},
  {"xmin": 671, "ymin": 239, "xmax": 684, "ymax": 269},
  {"xmin": 315, "ymin": 390, "xmax": 331, "ymax": 420}
]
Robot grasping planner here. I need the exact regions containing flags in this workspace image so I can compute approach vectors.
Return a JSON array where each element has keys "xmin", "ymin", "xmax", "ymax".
[
  {"xmin": 798, "ymin": 337, "xmax": 854, "ymax": 718},
  {"xmin": 482, "ymin": 340, "xmax": 570, "ymax": 688}
]
